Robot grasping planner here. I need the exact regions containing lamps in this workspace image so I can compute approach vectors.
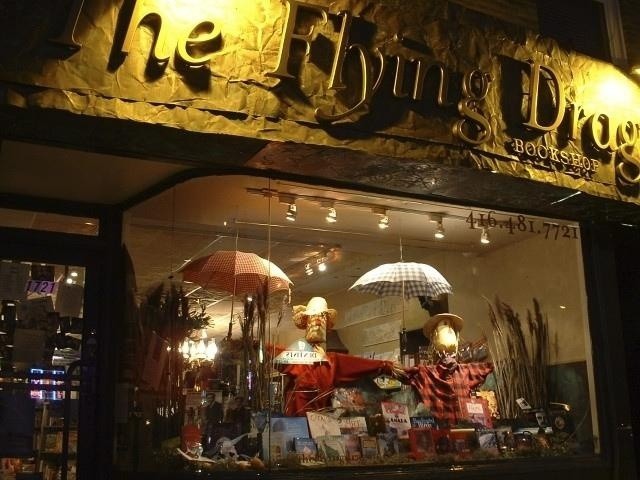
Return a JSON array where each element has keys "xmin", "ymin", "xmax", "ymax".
[
  {"xmin": 305, "ymin": 262, "xmax": 313, "ymax": 275},
  {"xmin": 278, "ymin": 196, "xmax": 297, "ymax": 221},
  {"xmin": 318, "ymin": 253, "xmax": 327, "ymax": 272},
  {"xmin": 372, "ymin": 208, "xmax": 389, "ymax": 229},
  {"xmin": 475, "ymin": 222, "xmax": 491, "ymax": 243},
  {"xmin": 321, "ymin": 202, "xmax": 337, "ymax": 222},
  {"xmin": 430, "ymin": 215, "xmax": 446, "ymax": 239}
]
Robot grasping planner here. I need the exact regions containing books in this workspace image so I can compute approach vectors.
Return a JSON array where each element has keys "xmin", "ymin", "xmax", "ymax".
[
  {"xmin": 40, "ymin": 459, "xmax": 76, "ymax": 480},
  {"xmin": 459, "ymin": 397, "xmax": 493, "ymax": 429},
  {"xmin": 340, "ymin": 416, "xmax": 368, "ymax": 462},
  {"xmin": 49, "ymin": 416, "xmax": 66, "ymax": 426},
  {"xmin": 306, "ymin": 412, "xmax": 341, "ymax": 444},
  {"xmin": 33, "ymin": 409, "xmax": 43, "ymax": 429},
  {"xmin": 492, "ymin": 426, "xmax": 517, "ymax": 453},
  {"xmin": 410, "ymin": 417, "xmax": 437, "ymax": 430},
  {"xmin": 33, "ymin": 431, "xmax": 43, "ymax": 453},
  {"xmin": 318, "ymin": 435, "xmax": 346, "ymax": 460},
  {"xmin": 434, "ymin": 426, "xmax": 453, "ymax": 456},
  {"xmin": 271, "ymin": 417, "xmax": 310, "ymax": 451},
  {"xmin": 43, "ymin": 431, "xmax": 78, "ymax": 452},
  {"xmin": 331, "ymin": 388, "xmax": 367, "ymax": 416},
  {"xmin": 258, "ymin": 432, "xmax": 288, "ymax": 463},
  {"xmin": 360, "ymin": 436, "xmax": 379, "ymax": 458},
  {"xmin": 294, "ymin": 437, "xmax": 317, "ymax": 460},
  {"xmin": 476, "ymin": 391, "xmax": 501, "ymax": 420},
  {"xmin": 409, "ymin": 429, "xmax": 437, "ymax": 461},
  {"xmin": 0, "ymin": 457, "xmax": 37, "ymax": 480},
  {"xmin": 449, "ymin": 428, "xmax": 475, "ymax": 453},
  {"xmin": 381, "ymin": 401, "xmax": 411, "ymax": 439},
  {"xmin": 478, "ymin": 432, "xmax": 499, "ymax": 455}
]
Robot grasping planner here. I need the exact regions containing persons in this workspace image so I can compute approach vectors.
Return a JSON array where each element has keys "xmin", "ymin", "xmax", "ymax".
[
  {"xmin": 202, "ymin": 393, "xmax": 224, "ymax": 458},
  {"xmin": 387, "ymin": 313, "xmax": 503, "ymax": 429},
  {"xmin": 226, "ymin": 397, "xmax": 250, "ymax": 456},
  {"xmin": 222, "ymin": 297, "xmax": 409, "ymax": 420}
]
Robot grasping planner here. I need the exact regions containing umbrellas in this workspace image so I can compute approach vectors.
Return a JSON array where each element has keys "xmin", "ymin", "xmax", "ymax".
[
  {"xmin": 178, "ymin": 251, "xmax": 294, "ymax": 344},
  {"xmin": 347, "ymin": 261, "xmax": 453, "ymax": 344}
]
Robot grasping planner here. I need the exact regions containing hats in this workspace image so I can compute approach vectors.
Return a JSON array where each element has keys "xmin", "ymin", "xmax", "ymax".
[
  {"xmin": 303, "ymin": 297, "xmax": 328, "ymax": 315},
  {"xmin": 423, "ymin": 313, "xmax": 464, "ymax": 339}
]
